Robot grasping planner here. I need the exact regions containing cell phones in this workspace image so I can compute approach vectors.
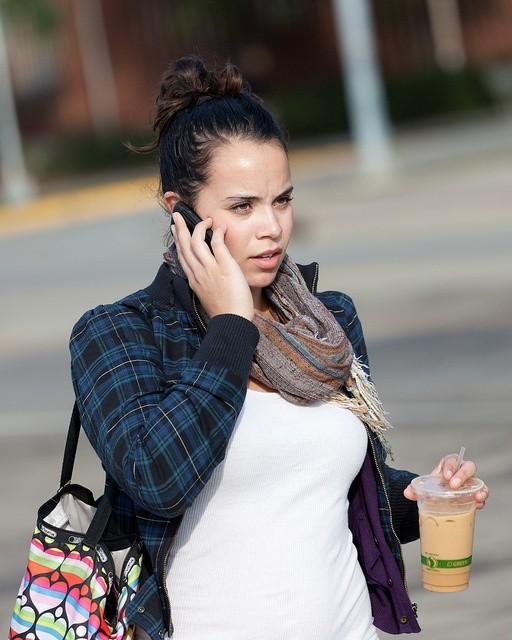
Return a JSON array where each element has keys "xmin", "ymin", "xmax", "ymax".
[{"xmin": 169, "ymin": 199, "xmax": 214, "ymax": 256}]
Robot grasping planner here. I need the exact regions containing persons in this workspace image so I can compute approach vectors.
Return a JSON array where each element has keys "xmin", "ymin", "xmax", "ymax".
[{"xmin": 67, "ymin": 56, "xmax": 490, "ymax": 639}]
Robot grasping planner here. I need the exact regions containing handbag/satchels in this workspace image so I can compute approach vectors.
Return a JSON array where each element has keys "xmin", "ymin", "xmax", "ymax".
[{"xmin": 9, "ymin": 304, "xmax": 154, "ymax": 639}]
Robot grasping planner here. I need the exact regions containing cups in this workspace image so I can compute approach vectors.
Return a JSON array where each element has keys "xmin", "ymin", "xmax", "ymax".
[{"xmin": 411, "ymin": 446, "xmax": 485, "ymax": 592}]
